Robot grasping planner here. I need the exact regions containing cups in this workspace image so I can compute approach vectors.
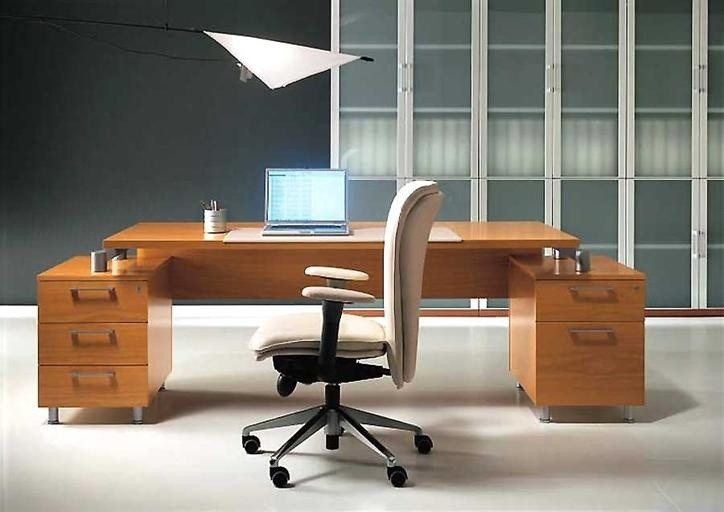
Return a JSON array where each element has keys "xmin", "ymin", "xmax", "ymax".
[
  {"xmin": 203, "ymin": 208, "xmax": 228, "ymax": 233},
  {"xmin": 575, "ymin": 249, "xmax": 591, "ymax": 272},
  {"xmin": 90, "ymin": 251, "xmax": 108, "ymax": 272}
]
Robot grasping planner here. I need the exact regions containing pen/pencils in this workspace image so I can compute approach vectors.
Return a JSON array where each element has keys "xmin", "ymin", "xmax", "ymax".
[{"xmin": 201, "ymin": 199, "xmax": 217, "ymax": 211}]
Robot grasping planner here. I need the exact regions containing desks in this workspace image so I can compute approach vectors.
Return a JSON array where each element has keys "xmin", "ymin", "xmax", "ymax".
[{"xmin": 102, "ymin": 221, "xmax": 580, "ymax": 391}]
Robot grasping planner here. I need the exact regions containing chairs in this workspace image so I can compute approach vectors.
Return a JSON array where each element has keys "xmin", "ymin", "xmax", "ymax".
[{"xmin": 242, "ymin": 181, "xmax": 444, "ymax": 487}]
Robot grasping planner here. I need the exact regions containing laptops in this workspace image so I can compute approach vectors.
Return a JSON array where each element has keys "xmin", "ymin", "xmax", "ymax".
[{"xmin": 261, "ymin": 167, "xmax": 350, "ymax": 236}]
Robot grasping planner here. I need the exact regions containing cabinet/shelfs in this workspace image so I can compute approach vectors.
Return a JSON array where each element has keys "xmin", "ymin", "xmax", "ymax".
[
  {"xmin": 35, "ymin": 253, "xmax": 173, "ymax": 425},
  {"xmin": 506, "ymin": 253, "xmax": 645, "ymax": 423}
]
